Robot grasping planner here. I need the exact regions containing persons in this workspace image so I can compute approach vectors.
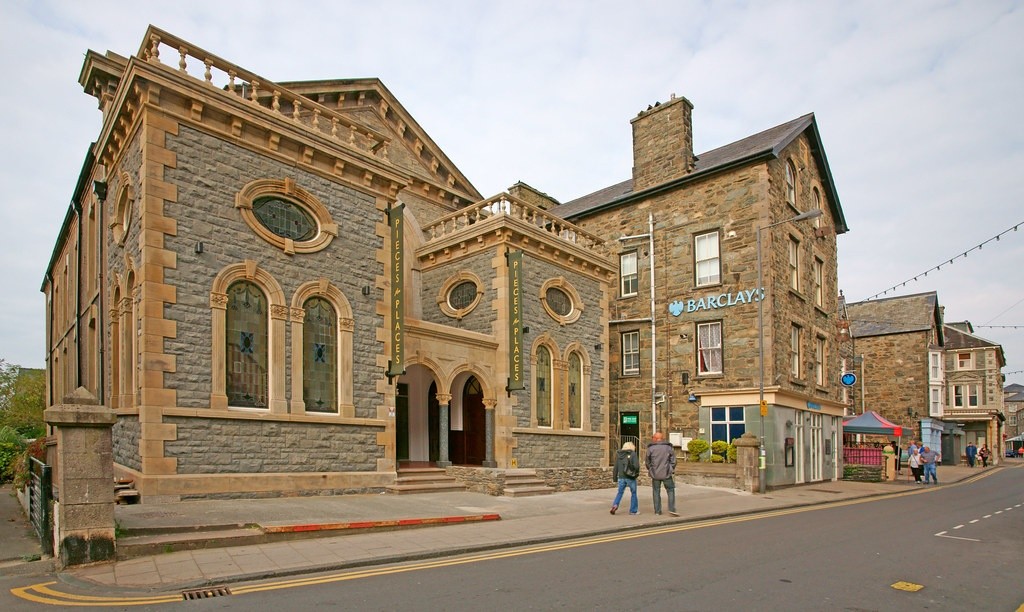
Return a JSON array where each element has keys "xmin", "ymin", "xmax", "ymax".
[
  {"xmin": 645, "ymin": 432, "xmax": 681, "ymax": 517},
  {"xmin": 915, "ymin": 441, "xmax": 925, "ymax": 478},
  {"xmin": 908, "ymin": 440, "xmax": 917, "ymax": 476},
  {"xmin": 1014, "ymin": 446, "xmax": 1024, "ymax": 458},
  {"xmin": 907, "ymin": 449, "xmax": 921, "ymax": 484},
  {"xmin": 610, "ymin": 442, "xmax": 642, "ymax": 515},
  {"xmin": 890, "ymin": 441, "xmax": 903, "ymax": 475},
  {"xmin": 920, "ymin": 446, "xmax": 940, "ymax": 485},
  {"xmin": 979, "ymin": 444, "xmax": 992, "ymax": 468},
  {"xmin": 965, "ymin": 442, "xmax": 977, "ymax": 468}
]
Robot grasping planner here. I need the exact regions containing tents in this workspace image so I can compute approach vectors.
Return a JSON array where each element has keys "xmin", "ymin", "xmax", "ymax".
[{"xmin": 843, "ymin": 411, "xmax": 915, "ymax": 479}]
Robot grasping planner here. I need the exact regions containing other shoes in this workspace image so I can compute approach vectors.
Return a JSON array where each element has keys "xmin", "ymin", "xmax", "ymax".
[
  {"xmin": 610, "ymin": 505, "xmax": 618, "ymax": 514},
  {"xmin": 934, "ymin": 480, "xmax": 937, "ymax": 484},
  {"xmin": 669, "ymin": 511, "xmax": 680, "ymax": 516},
  {"xmin": 915, "ymin": 480, "xmax": 920, "ymax": 484},
  {"xmin": 923, "ymin": 482, "xmax": 928, "ymax": 484},
  {"xmin": 630, "ymin": 512, "xmax": 640, "ymax": 515}
]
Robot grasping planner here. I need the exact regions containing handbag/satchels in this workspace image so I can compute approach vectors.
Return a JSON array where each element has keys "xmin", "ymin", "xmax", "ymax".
[
  {"xmin": 918, "ymin": 465, "xmax": 923, "ymax": 469},
  {"xmin": 624, "ymin": 452, "xmax": 639, "ymax": 480}
]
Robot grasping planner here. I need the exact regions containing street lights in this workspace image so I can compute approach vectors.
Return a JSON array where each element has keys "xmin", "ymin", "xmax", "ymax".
[{"xmin": 755, "ymin": 209, "xmax": 825, "ymax": 495}]
